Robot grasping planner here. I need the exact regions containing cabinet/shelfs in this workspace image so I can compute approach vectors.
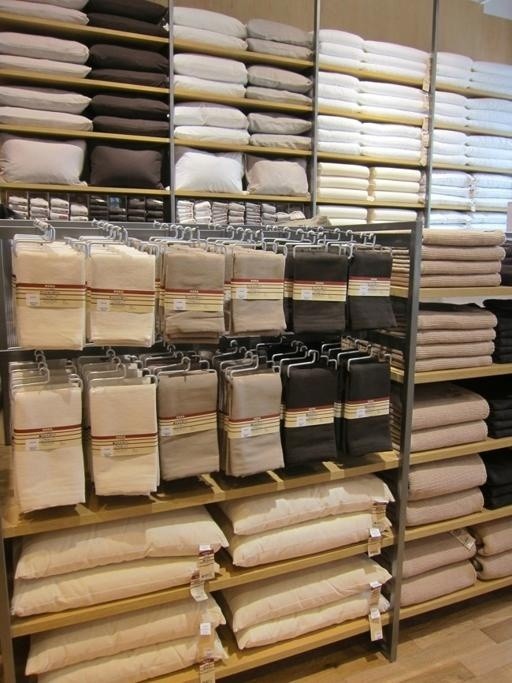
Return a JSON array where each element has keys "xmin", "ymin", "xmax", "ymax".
[
  {"xmin": 393, "ymin": 219, "xmax": 512, "ymax": 665},
  {"xmin": 1, "ymin": 216, "xmax": 422, "ymax": 679},
  {"xmin": 2, "ymin": 0, "xmax": 511, "ymax": 230}
]
